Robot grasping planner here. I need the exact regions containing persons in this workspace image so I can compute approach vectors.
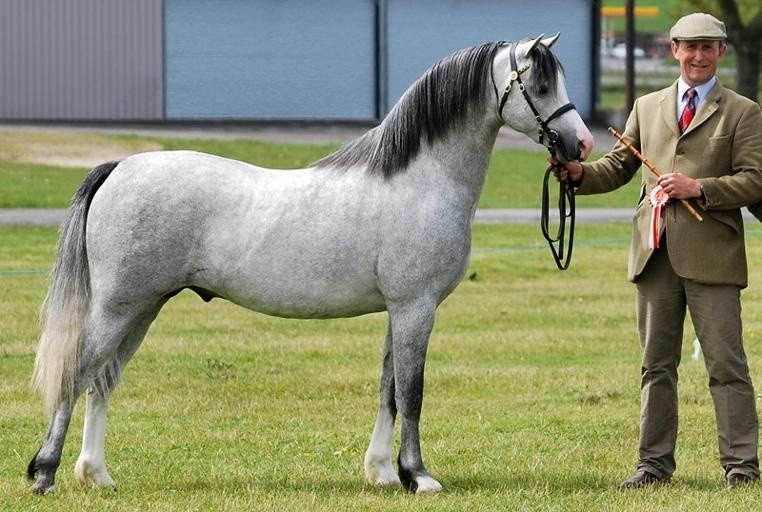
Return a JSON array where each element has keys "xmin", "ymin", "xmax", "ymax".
[{"xmin": 551, "ymin": 13, "xmax": 762, "ymax": 491}]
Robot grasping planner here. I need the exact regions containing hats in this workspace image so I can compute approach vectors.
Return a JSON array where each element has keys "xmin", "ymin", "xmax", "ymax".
[{"xmin": 668, "ymin": 12, "xmax": 729, "ymax": 42}]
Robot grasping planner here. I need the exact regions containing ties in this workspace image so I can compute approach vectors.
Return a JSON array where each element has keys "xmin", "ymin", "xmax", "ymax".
[{"xmin": 678, "ymin": 88, "xmax": 698, "ymax": 135}]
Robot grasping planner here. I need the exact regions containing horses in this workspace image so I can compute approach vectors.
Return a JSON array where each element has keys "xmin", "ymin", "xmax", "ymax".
[{"xmin": 24, "ymin": 29, "xmax": 597, "ymax": 498}]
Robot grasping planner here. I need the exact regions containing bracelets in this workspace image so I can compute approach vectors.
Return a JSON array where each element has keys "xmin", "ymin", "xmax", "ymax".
[{"xmin": 700, "ymin": 185, "xmax": 704, "ymax": 199}]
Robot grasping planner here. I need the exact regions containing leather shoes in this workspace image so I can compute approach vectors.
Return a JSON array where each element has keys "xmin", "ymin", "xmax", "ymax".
[
  {"xmin": 727, "ymin": 474, "xmax": 752, "ymax": 489},
  {"xmin": 621, "ymin": 470, "xmax": 658, "ymax": 489}
]
